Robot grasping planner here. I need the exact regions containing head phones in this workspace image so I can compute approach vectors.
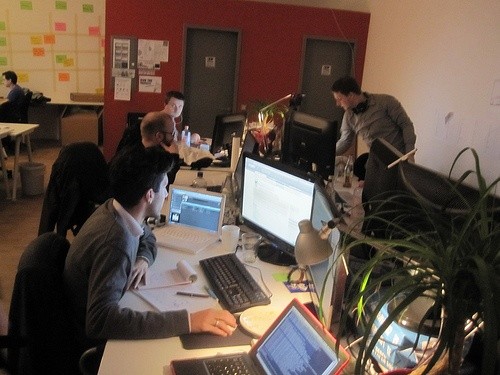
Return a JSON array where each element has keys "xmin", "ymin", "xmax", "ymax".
[{"xmin": 146, "ymin": 214, "xmax": 166, "ymax": 228}]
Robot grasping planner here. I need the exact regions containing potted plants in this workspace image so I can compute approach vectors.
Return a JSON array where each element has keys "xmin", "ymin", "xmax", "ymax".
[{"xmin": 319, "ymin": 147, "xmax": 500, "ymax": 375}]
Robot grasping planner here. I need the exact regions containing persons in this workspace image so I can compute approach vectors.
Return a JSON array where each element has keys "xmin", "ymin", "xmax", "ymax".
[
  {"xmin": 93, "ymin": 111, "xmax": 180, "ymax": 204},
  {"xmin": 0, "ymin": 70, "xmax": 27, "ymax": 154},
  {"xmin": 331, "ymin": 76, "xmax": 416, "ymax": 178},
  {"xmin": 160, "ymin": 91, "xmax": 201, "ymax": 144},
  {"xmin": 60, "ymin": 147, "xmax": 238, "ymax": 364}
]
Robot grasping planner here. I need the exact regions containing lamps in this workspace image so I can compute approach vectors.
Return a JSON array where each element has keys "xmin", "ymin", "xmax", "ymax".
[{"xmin": 294, "ymin": 199, "xmax": 436, "ymax": 267}]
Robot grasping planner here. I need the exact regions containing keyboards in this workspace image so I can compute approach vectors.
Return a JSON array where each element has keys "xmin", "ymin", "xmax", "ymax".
[{"xmin": 199, "ymin": 252, "xmax": 271, "ymax": 313}]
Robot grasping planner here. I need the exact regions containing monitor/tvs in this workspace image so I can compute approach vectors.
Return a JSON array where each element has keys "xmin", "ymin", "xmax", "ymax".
[
  {"xmin": 211, "ymin": 111, "xmax": 247, "ymax": 166},
  {"xmin": 282, "ymin": 109, "xmax": 337, "ymax": 179},
  {"xmin": 240, "ymin": 151, "xmax": 316, "ymax": 265}
]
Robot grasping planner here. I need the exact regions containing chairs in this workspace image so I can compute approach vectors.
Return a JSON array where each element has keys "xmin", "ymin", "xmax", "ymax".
[
  {"xmin": 0, "ymin": 91, "xmax": 34, "ymax": 156},
  {"xmin": 38, "ymin": 142, "xmax": 109, "ymax": 240},
  {"xmin": 0, "ymin": 232, "xmax": 100, "ymax": 375}
]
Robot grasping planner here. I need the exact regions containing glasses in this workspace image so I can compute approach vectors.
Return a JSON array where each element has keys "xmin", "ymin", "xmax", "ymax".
[{"xmin": 161, "ymin": 130, "xmax": 176, "ymax": 136}]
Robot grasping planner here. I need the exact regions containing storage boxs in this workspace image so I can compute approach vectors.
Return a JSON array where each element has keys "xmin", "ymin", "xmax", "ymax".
[{"xmin": 56, "ymin": 105, "xmax": 104, "ymax": 146}]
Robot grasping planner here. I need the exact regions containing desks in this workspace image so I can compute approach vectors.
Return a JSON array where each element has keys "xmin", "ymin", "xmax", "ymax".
[
  {"xmin": 46, "ymin": 98, "xmax": 104, "ymax": 146},
  {"xmin": 0, "ymin": 123, "xmax": 39, "ymax": 203},
  {"xmin": 97, "ymin": 137, "xmax": 359, "ymax": 375}
]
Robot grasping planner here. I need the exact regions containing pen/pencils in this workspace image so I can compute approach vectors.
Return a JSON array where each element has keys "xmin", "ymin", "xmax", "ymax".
[
  {"xmin": 176, "ymin": 291, "xmax": 209, "ymax": 298},
  {"xmin": 204, "ymin": 283, "xmax": 219, "ymax": 302}
]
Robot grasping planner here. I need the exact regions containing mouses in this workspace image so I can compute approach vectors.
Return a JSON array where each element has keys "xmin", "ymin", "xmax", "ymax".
[{"xmin": 224, "ymin": 322, "xmax": 237, "ymax": 331}]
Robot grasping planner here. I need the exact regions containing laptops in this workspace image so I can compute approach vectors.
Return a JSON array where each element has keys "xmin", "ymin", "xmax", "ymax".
[
  {"xmin": 151, "ymin": 184, "xmax": 226, "ymax": 254},
  {"xmin": 171, "ymin": 298, "xmax": 351, "ymax": 375}
]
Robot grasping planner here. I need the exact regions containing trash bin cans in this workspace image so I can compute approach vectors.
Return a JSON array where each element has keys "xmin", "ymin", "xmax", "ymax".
[{"xmin": 19, "ymin": 161, "xmax": 45, "ymax": 196}]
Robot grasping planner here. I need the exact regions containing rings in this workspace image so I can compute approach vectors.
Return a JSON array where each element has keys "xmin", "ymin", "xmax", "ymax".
[{"xmin": 214, "ymin": 320, "xmax": 219, "ymax": 326}]
